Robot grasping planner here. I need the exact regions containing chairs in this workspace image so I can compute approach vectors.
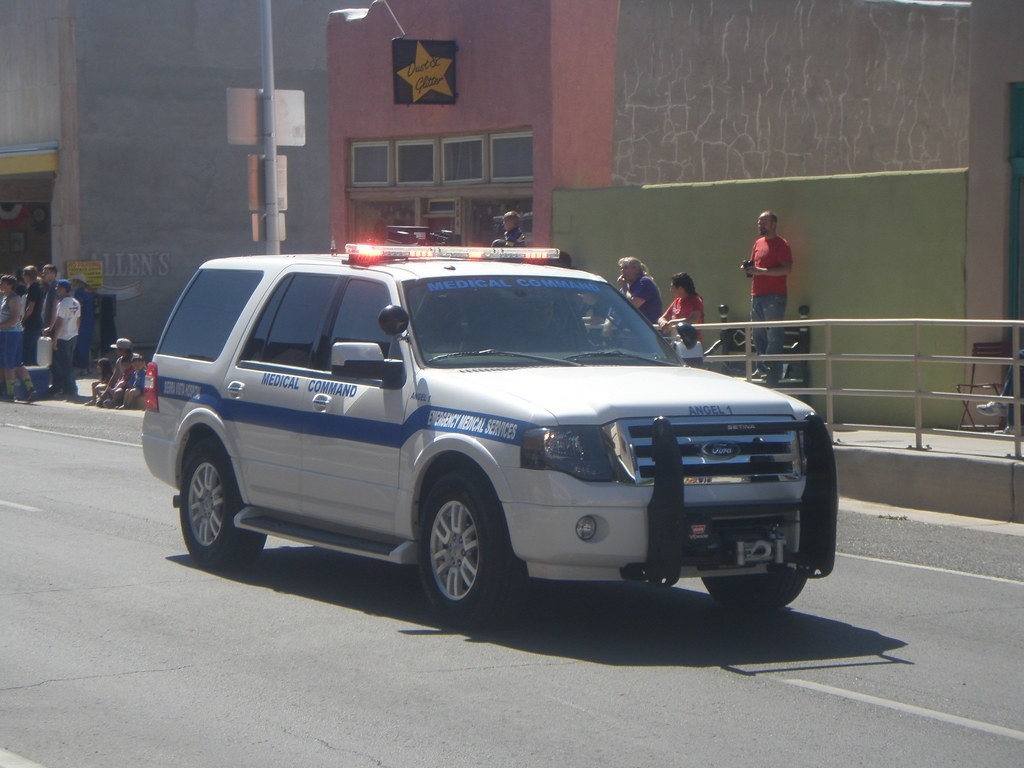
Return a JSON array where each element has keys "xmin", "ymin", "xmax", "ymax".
[{"xmin": 956, "ymin": 342, "xmax": 1013, "ymax": 433}]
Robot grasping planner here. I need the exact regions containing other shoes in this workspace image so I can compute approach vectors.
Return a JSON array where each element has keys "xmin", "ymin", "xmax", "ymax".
[
  {"xmin": 49, "ymin": 386, "xmax": 76, "ymax": 396},
  {"xmin": 84, "ymin": 399, "xmax": 130, "ymax": 409},
  {"xmin": 752, "ymin": 369, "xmax": 764, "ymax": 379},
  {"xmin": 0, "ymin": 393, "xmax": 15, "ymax": 402},
  {"xmin": 762, "ymin": 373, "xmax": 767, "ymax": 378},
  {"xmin": 25, "ymin": 389, "xmax": 37, "ymax": 400}
]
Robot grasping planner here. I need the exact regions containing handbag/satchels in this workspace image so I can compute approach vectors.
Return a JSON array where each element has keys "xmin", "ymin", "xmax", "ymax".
[{"xmin": 36, "ymin": 334, "xmax": 53, "ymax": 367}]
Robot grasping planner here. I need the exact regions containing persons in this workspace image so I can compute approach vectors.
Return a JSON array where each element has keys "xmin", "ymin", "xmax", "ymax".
[
  {"xmin": 547, "ymin": 251, "xmax": 572, "ymax": 269},
  {"xmin": 576, "ymin": 293, "xmax": 610, "ymax": 346},
  {"xmin": 0, "ymin": 264, "xmax": 58, "ymax": 402},
  {"xmin": 657, "ymin": 272, "xmax": 704, "ymax": 344},
  {"xmin": 601, "ymin": 256, "xmax": 671, "ymax": 344},
  {"xmin": 97, "ymin": 337, "xmax": 140, "ymax": 408},
  {"xmin": 490, "ymin": 211, "xmax": 527, "ymax": 263},
  {"xmin": 739, "ymin": 211, "xmax": 794, "ymax": 379},
  {"xmin": 53, "ymin": 279, "xmax": 81, "ymax": 401},
  {"xmin": 115, "ymin": 355, "xmax": 146, "ymax": 410},
  {"xmin": 84, "ymin": 358, "xmax": 119, "ymax": 406}
]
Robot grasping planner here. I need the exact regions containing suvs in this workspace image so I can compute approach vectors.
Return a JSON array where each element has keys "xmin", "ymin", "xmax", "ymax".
[{"xmin": 141, "ymin": 242, "xmax": 838, "ymax": 630}]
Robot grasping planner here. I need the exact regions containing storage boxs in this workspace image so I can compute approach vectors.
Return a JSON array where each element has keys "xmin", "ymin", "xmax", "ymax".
[{"xmin": 15, "ymin": 336, "xmax": 53, "ymax": 401}]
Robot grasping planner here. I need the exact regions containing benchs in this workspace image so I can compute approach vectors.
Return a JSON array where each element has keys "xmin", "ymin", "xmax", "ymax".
[{"xmin": 704, "ymin": 304, "xmax": 811, "ymax": 406}]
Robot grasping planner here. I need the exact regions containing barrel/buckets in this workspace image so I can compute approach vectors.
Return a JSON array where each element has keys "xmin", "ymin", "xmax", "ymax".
[{"xmin": 35, "ymin": 334, "xmax": 53, "ymax": 366}]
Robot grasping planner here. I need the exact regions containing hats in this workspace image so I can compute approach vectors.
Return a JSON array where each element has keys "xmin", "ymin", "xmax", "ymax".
[
  {"xmin": 72, "ymin": 273, "xmax": 88, "ymax": 286},
  {"xmin": 58, "ymin": 278, "xmax": 73, "ymax": 292},
  {"xmin": 110, "ymin": 338, "xmax": 131, "ymax": 349}
]
